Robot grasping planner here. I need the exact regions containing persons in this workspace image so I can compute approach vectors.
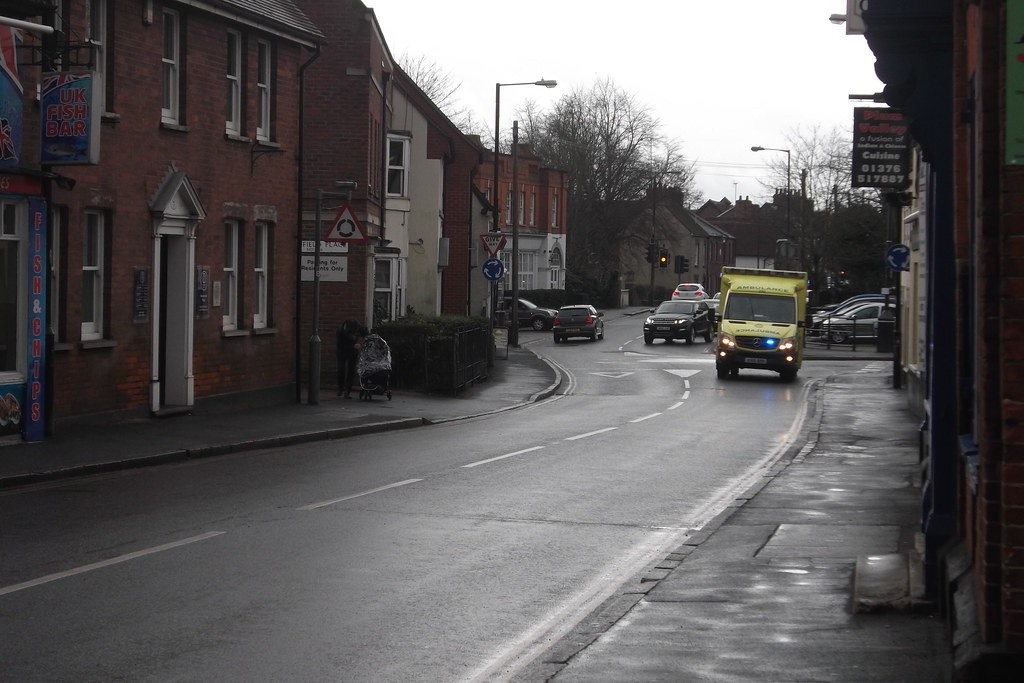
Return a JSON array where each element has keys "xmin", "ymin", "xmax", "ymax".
[{"xmin": 336, "ymin": 317, "xmax": 370, "ymax": 399}]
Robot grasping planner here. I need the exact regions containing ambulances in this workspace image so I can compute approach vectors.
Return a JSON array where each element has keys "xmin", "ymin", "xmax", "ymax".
[{"xmin": 716, "ymin": 266, "xmax": 805, "ymax": 382}]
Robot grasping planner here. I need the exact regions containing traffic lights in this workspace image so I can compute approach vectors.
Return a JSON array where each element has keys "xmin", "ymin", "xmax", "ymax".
[
  {"xmin": 646, "ymin": 244, "xmax": 656, "ymax": 263},
  {"xmin": 658, "ymin": 249, "xmax": 669, "ymax": 269},
  {"xmin": 675, "ymin": 255, "xmax": 689, "ymax": 273}
]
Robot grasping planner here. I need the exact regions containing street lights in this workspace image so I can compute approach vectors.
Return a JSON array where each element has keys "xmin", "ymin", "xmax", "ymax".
[
  {"xmin": 492, "ymin": 78, "xmax": 557, "ymax": 325},
  {"xmin": 645, "ymin": 171, "xmax": 682, "ymax": 305},
  {"xmin": 752, "ymin": 147, "xmax": 791, "ymax": 270}
]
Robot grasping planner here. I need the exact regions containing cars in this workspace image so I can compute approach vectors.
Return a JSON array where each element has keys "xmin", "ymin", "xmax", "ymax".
[
  {"xmin": 553, "ymin": 304, "xmax": 604, "ymax": 341},
  {"xmin": 671, "ymin": 282, "xmax": 709, "ymax": 300},
  {"xmin": 821, "ymin": 302, "xmax": 896, "ymax": 343},
  {"xmin": 713, "ymin": 291, "xmax": 722, "ymax": 320},
  {"xmin": 807, "ymin": 293, "xmax": 896, "ymax": 336},
  {"xmin": 504, "ymin": 296, "xmax": 558, "ymax": 330}
]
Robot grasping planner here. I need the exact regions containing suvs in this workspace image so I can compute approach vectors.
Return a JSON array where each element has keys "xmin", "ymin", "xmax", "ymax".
[{"xmin": 644, "ymin": 300, "xmax": 714, "ymax": 344}]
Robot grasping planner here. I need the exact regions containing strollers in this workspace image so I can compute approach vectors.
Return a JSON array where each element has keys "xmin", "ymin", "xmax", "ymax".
[{"xmin": 357, "ymin": 334, "xmax": 393, "ymax": 400}]
